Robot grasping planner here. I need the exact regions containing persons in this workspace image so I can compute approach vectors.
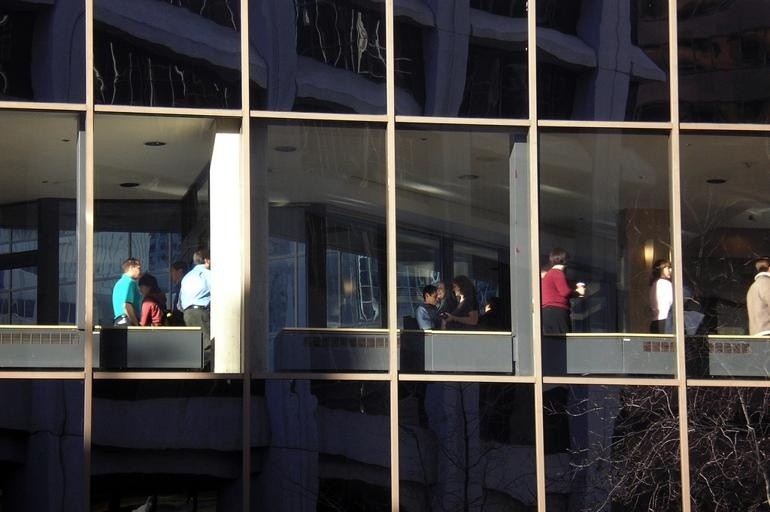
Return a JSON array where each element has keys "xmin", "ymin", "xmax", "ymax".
[
  {"xmin": 109, "ymin": 256, "xmax": 142, "ymax": 329},
  {"xmin": 481, "ymin": 296, "xmax": 500, "ymax": 316},
  {"xmin": 540, "ymin": 267, "xmax": 551, "ymax": 281},
  {"xmin": 177, "ymin": 250, "xmax": 214, "ymax": 369},
  {"xmin": 746, "ymin": 256, "xmax": 770, "ymax": 339},
  {"xmin": 542, "ymin": 249, "xmax": 587, "ymax": 334},
  {"xmin": 167, "ymin": 258, "xmax": 193, "ymax": 328},
  {"xmin": 438, "ymin": 274, "xmax": 478, "ymax": 330},
  {"xmin": 415, "ymin": 287, "xmax": 442, "ymax": 333},
  {"xmin": 136, "ymin": 276, "xmax": 165, "ymax": 326},
  {"xmin": 644, "ymin": 257, "xmax": 675, "ymax": 333},
  {"xmin": 434, "ymin": 281, "xmax": 458, "ymax": 314}
]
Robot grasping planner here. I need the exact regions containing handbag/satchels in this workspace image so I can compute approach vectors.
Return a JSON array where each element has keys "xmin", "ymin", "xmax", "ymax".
[{"xmin": 164, "ymin": 309, "xmax": 180, "ymax": 323}]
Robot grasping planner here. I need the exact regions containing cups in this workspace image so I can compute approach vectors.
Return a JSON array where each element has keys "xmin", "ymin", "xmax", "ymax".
[{"xmin": 575, "ymin": 281, "xmax": 585, "ymax": 299}]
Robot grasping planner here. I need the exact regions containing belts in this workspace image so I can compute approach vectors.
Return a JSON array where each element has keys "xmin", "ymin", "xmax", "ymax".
[{"xmin": 188, "ymin": 305, "xmax": 209, "ymax": 311}]
